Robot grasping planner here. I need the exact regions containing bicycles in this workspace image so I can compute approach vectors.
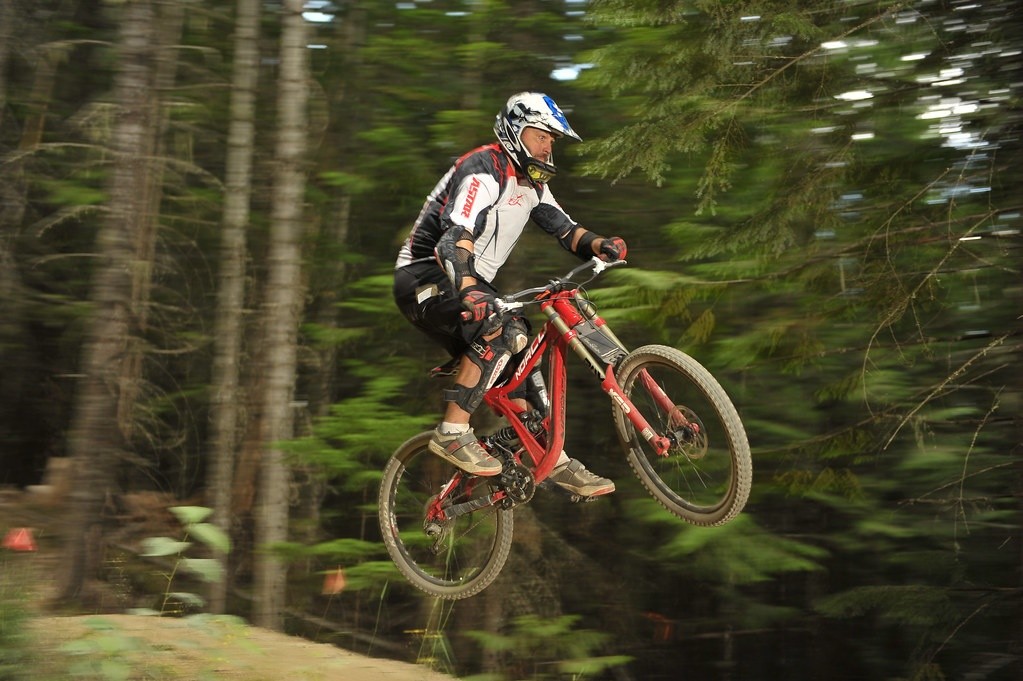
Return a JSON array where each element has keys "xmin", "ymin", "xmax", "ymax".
[{"xmin": 377, "ymin": 256, "xmax": 752, "ymax": 600}]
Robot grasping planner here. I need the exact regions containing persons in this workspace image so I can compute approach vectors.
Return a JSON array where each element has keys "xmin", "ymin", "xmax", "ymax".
[{"xmin": 392, "ymin": 89, "xmax": 629, "ymax": 499}]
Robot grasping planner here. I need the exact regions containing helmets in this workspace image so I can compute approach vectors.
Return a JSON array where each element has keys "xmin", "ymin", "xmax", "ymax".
[{"xmin": 493, "ymin": 91, "xmax": 583, "ymax": 183}]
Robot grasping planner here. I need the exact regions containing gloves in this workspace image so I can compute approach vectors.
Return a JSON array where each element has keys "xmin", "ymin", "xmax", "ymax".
[
  {"xmin": 459, "ymin": 286, "xmax": 504, "ymax": 326},
  {"xmin": 601, "ymin": 238, "xmax": 626, "ymax": 264}
]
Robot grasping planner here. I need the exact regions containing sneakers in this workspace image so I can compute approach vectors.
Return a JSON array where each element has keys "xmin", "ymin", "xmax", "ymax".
[
  {"xmin": 548, "ymin": 457, "xmax": 615, "ymax": 498},
  {"xmin": 427, "ymin": 424, "xmax": 503, "ymax": 477}
]
